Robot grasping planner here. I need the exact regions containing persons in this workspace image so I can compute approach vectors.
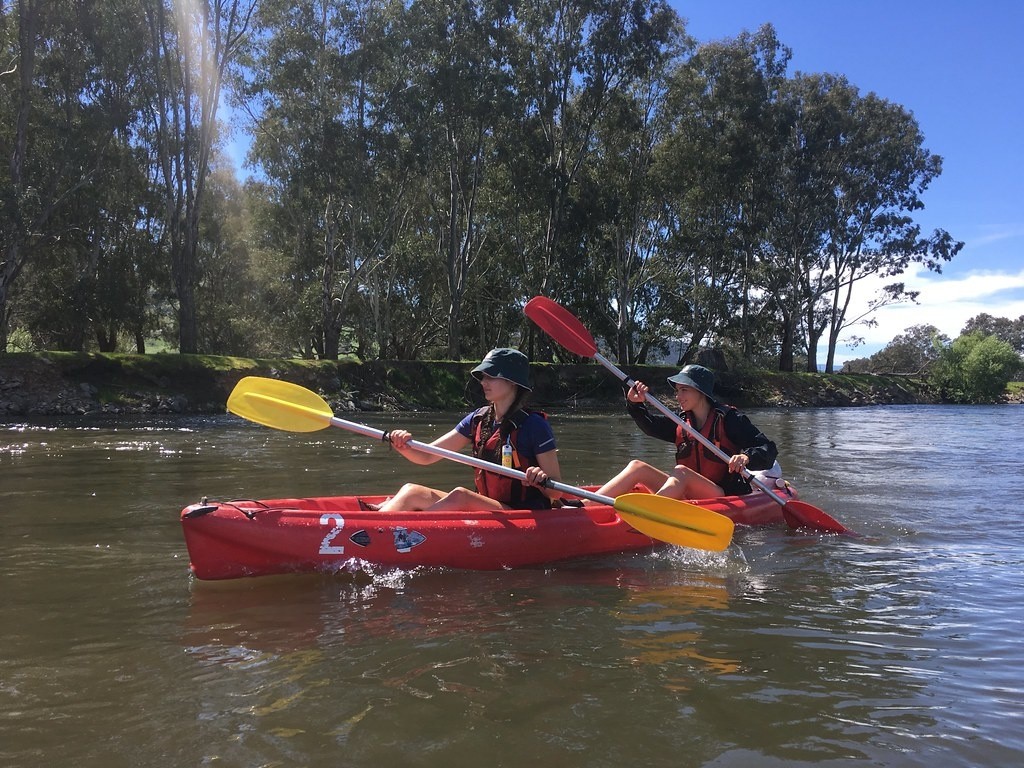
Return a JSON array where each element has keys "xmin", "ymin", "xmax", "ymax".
[
  {"xmin": 377, "ymin": 347, "xmax": 560, "ymax": 511},
  {"xmin": 559, "ymin": 364, "xmax": 778, "ymax": 507}
]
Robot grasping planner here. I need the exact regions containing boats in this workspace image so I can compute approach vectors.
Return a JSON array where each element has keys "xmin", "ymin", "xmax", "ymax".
[{"xmin": 180, "ymin": 485, "xmax": 798, "ymax": 581}]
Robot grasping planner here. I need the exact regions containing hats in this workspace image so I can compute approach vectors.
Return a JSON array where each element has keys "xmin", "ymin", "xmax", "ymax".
[
  {"xmin": 666, "ymin": 364, "xmax": 715, "ymax": 403},
  {"xmin": 470, "ymin": 348, "xmax": 533, "ymax": 393}
]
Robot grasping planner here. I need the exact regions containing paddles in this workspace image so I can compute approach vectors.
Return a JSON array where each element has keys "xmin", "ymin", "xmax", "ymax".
[
  {"xmin": 225, "ymin": 375, "xmax": 735, "ymax": 554},
  {"xmin": 522, "ymin": 295, "xmax": 848, "ymax": 532}
]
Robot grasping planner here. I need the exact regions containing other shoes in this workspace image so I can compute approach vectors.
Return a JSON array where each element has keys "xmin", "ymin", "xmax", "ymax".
[
  {"xmin": 358, "ymin": 498, "xmax": 382, "ymax": 511},
  {"xmin": 558, "ymin": 497, "xmax": 585, "ymax": 507}
]
